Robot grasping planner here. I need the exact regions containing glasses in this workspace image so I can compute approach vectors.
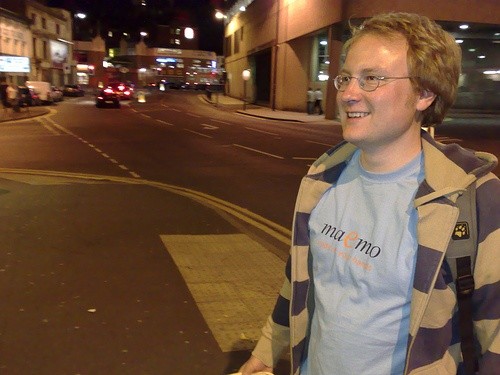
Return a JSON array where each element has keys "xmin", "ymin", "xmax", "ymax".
[{"xmin": 331, "ymin": 74, "xmax": 412, "ymax": 93}]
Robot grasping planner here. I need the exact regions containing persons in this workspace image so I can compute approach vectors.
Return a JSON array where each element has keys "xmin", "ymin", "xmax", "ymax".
[
  {"xmin": 238, "ymin": 11, "xmax": 500, "ymax": 375},
  {"xmin": 314, "ymin": 87, "xmax": 323, "ymax": 115},
  {"xmin": 306, "ymin": 87, "xmax": 315, "ymax": 115}
]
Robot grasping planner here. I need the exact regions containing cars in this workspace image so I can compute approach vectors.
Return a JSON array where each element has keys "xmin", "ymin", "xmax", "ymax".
[
  {"xmin": 96, "ymin": 82, "xmax": 133, "ymax": 108},
  {"xmin": 63, "ymin": 84, "xmax": 85, "ymax": 97},
  {"xmin": 0, "ymin": 84, "xmax": 42, "ymax": 106}
]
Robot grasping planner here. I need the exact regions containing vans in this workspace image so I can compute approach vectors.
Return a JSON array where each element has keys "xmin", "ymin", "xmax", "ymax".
[{"xmin": 23, "ymin": 81, "xmax": 63, "ymax": 104}]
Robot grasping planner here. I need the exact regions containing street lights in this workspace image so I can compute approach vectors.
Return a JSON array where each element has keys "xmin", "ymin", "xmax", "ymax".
[{"xmin": 74, "ymin": 12, "xmax": 86, "ymax": 61}]
